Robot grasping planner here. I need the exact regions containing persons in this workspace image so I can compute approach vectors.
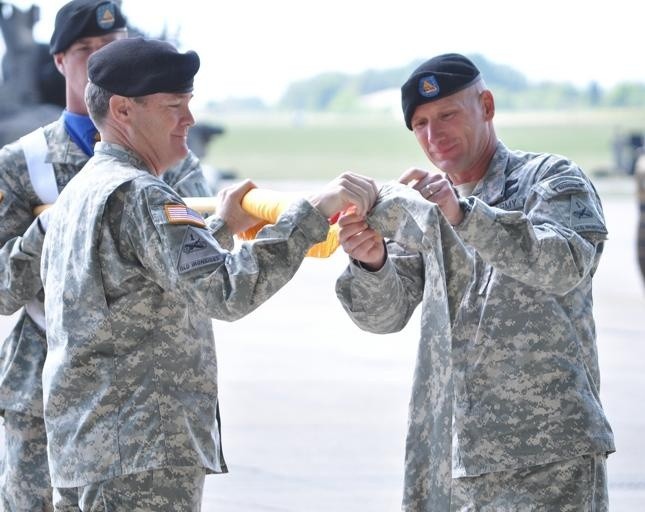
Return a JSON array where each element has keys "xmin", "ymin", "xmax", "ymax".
[
  {"xmin": 330, "ymin": 53, "xmax": 619, "ymax": 511},
  {"xmin": 0, "ymin": 0, "xmax": 214, "ymax": 510},
  {"xmin": 37, "ymin": 35, "xmax": 382, "ymax": 511}
]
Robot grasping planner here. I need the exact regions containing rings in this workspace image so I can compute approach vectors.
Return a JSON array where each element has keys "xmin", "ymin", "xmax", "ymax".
[{"xmin": 425, "ymin": 184, "xmax": 433, "ymax": 196}]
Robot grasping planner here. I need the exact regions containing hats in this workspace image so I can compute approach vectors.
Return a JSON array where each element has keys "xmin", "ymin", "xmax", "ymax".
[
  {"xmin": 48, "ymin": 0, "xmax": 128, "ymax": 54},
  {"xmin": 400, "ymin": 52, "xmax": 484, "ymax": 131},
  {"xmin": 86, "ymin": 37, "xmax": 202, "ymax": 98}
]
